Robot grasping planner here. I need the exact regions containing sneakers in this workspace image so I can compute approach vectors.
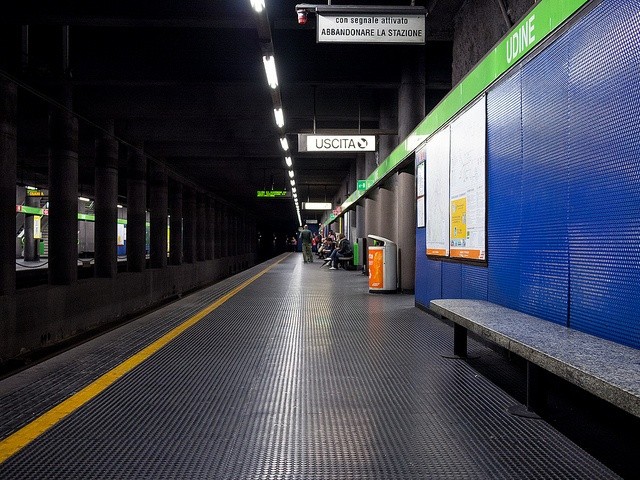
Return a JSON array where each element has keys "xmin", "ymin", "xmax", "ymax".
[
  {"xmin": 329, "ymin": 267, "xmax": 336, "ymax": 269},
  {"xmin": 324, "ymin": 257, "xmax": 332, "ymax": 261}
]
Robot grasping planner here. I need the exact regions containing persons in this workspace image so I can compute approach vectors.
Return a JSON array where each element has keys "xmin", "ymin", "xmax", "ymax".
[
  {"xmin": 324, "ymin": 234, "xmax": 353, "ymax": 270},
  {"xmin": 312, "ymin": 232, "xmax": 335, "ymax": 258},
  {"xmin": 299, "ymin": 224, "xmax": 313, "ymax": 263}
]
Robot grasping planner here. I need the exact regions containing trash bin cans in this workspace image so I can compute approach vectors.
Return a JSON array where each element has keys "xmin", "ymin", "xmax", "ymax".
[{"xmin": 367, "ymin": 234, "xmax": 397, "ymax": 293}]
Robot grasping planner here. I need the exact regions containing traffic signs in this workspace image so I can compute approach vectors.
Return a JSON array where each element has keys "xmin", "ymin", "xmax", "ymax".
[
  {"xmin": 315, "ymin": 13, "xmax": 428, "ymax": 44},
  {"xmin": 298, "ymin": 132, "xmax": 378, "ymax": 153}
]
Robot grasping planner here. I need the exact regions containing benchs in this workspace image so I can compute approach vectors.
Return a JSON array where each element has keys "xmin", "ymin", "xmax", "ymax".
[
  {"xmin": 338, "ymin": 256, "xmax": 354, "ymax": 270},
  {"xmin": 431, "ymin": 298, "xmax": 640, "ymax": 412}
]
